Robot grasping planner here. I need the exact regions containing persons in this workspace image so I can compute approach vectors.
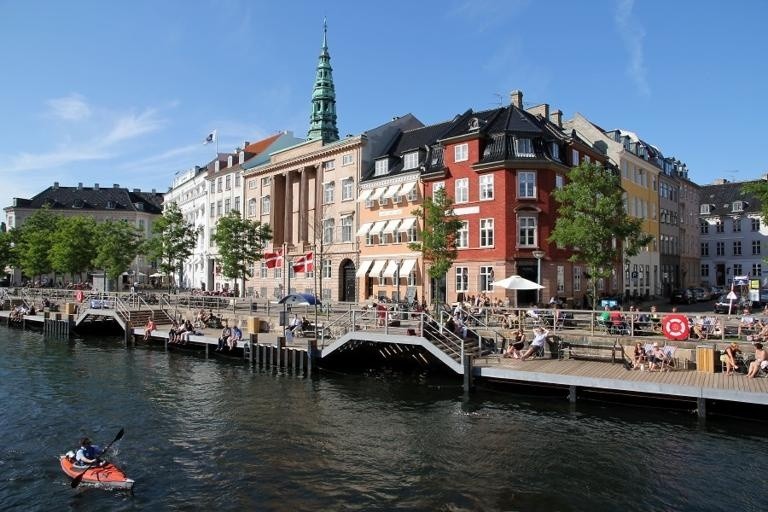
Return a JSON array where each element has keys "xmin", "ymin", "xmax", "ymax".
[
  {"xmin": 505, "ymin": 327, "xmax": 549, "ymax": 361},
  {"xmin": 361, "ymin": 294, "xmax": 566, "ymax": 340},
  {"xmin": 75, "ymin": 434, "xmax": 108, "ymax": 466},
  {"xmin": 632, "ymin": 342, "xmax": 669, "ymax": 372},
  {"xmin": 168, "ymin": 308, "xmax": 242, "ymax": 351},
  {"xmin": 26, "ymin": 277, "xmax": 89, "ymax": 290},
  {"xmin": 599, "ymin": 303, "xmax": 768, "ymax": 341},
  {"xmin": 143, "ymin": 316, "xmax": 156, "ymax": 341},
  {"xmin": 0, "ymin": 296, "xmax": 50, "ymax": 323},
  {"xmin": 719, "ymin": 342, "xmax": 768, "ymax": 379},
  {"xmin": 284, "ymin": 314, "xmax": 310, "ymax": 338}
]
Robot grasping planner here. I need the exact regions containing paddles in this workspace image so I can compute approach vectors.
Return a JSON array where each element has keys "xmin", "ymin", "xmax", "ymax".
[{"xmin": 71, "ymin": 427, "xmax": 124, "ymax": 489}]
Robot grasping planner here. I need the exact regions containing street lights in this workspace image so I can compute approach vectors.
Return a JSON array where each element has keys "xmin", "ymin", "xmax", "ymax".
[
  {"xmin": 395, "ymin": 260, "xmax": 403, "ymax": 304},
  {"xmin": 532, "ymin": 250, "xmax": 547, "ymax": 304}
]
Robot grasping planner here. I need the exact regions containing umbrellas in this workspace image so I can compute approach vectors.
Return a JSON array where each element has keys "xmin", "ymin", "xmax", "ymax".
[{"xmin": 488, "ymin": 275, "xmax": 545, "ymax": 308}]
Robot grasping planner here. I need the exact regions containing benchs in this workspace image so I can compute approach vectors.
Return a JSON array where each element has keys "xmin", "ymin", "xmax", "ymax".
[{"xmin": 300, "ymin": 323, "xmax": 323, "ymax": 337}]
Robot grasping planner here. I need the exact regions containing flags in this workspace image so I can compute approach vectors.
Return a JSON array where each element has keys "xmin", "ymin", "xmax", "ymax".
[
  {"xmin": 292, "ymin": 250, "xmax": 314, "ymax": 273},
  {"xmin": 264, "ymin": 245, "xmax": 286, "ymax": 268},
  {"xmin": 203, "ymin": 129, "xmax": 217, "ymax": 145}
]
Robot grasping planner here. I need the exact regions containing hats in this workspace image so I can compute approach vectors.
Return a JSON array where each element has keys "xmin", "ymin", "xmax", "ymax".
[{"xmin": 652, "ymin": 342, "xmax": 658, "ymax": 346}]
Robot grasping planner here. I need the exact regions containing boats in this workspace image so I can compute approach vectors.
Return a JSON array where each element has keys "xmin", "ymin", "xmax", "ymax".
[{"xmin": 59, "ymin": 450, "xmax": 136, "ymax": 490}]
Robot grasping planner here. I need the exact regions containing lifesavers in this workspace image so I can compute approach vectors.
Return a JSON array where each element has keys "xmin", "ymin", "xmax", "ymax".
[
  {"xmin": 666, "ymin": 318, "xmax": 685, "ymax": 337},
  {"xmin": 76, "ymin": 291, "xmax": 83, "ymax": 302}
]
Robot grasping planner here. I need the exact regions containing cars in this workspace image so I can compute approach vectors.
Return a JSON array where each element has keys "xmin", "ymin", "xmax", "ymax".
[
  {"xmin": 670, "ymin": 286, "xmax": 741, "ymax": 313},
  {"xmin": 761, "ymin": 289, "xmax": 768, "ymax": 302}
]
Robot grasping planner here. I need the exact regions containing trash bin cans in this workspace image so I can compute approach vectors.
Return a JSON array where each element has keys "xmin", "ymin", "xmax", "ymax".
[
  {"xmin": 696, "ymin": 345, "xmax": 716, "ymax": 373},
  {"xmin": 279, "ymin": 311, "xmax": 290, "ymax": 326},
  {"xmin": 400, "ymin": 305, "xmax": 408, "ymax": 320},
  {"xmin": 248, "ymin": 316, "xmax": 260, "ymax": 334},
  {"xmin": 66, "ymin": 302, "xmax": 74, "ymax": 314},
  {"xmin": 251, "ymin": 302, "xmax": 257, "ymax": 313},
  {"xmin": 565, "ymin": 297, "xmax": 574, "ymax": 309}
]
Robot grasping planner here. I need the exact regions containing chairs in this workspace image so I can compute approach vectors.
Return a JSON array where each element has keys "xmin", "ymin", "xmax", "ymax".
[
  {"xmin": 720, "ymin": 350, "xmax": 740, "ymax": 374},
  {"xmin": 632, "ymin": 343, "xmax": 678, "ymax": 373}
]
